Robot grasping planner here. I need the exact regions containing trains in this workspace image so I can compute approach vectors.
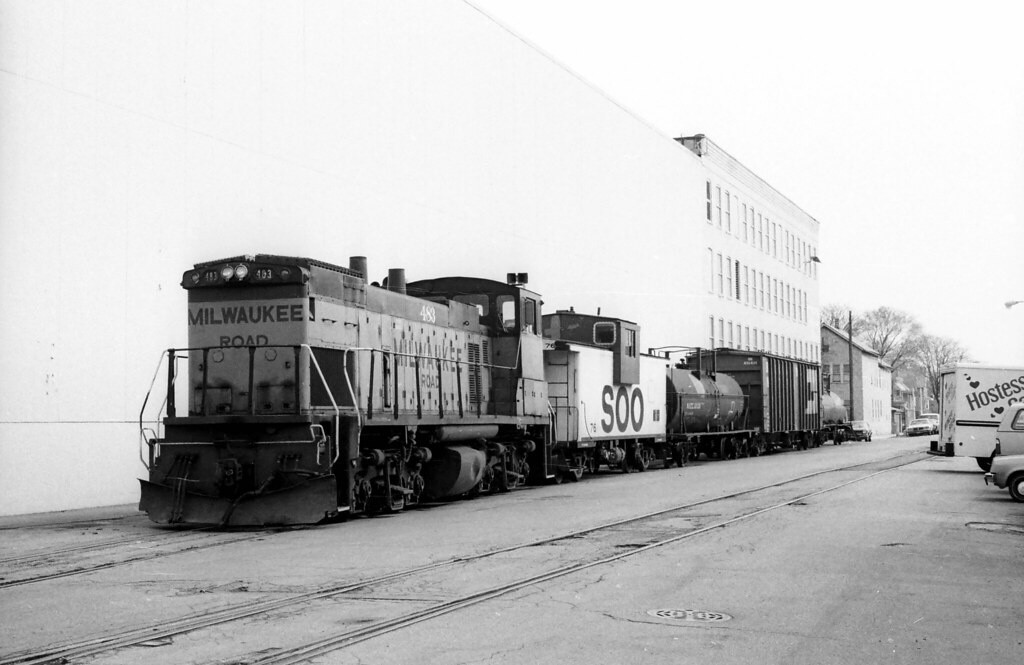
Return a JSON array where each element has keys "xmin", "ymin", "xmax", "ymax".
[{"xmin": 132, "ymin": 251, "xmax": 859, "ymax": 533}]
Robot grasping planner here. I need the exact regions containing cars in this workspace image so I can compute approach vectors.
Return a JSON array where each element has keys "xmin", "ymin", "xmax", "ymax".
[
  {"xmin": 907, "ymin": 413, "xmax": 939, "ymax": 437},
  {"xmin": 848, "ymin": 420, "xmax": 873, "ymax": 442}
]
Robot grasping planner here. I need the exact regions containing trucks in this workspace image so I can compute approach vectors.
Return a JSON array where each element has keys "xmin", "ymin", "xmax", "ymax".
[{"xmin": 925, "ymin": 361, "xmax": 1024, "ymax": 472}]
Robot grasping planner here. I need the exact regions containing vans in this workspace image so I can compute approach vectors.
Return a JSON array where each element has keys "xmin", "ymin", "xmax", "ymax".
[{"xmin": 983, "ymin": 404, "xmax": 1024, "ymax": 503}]
